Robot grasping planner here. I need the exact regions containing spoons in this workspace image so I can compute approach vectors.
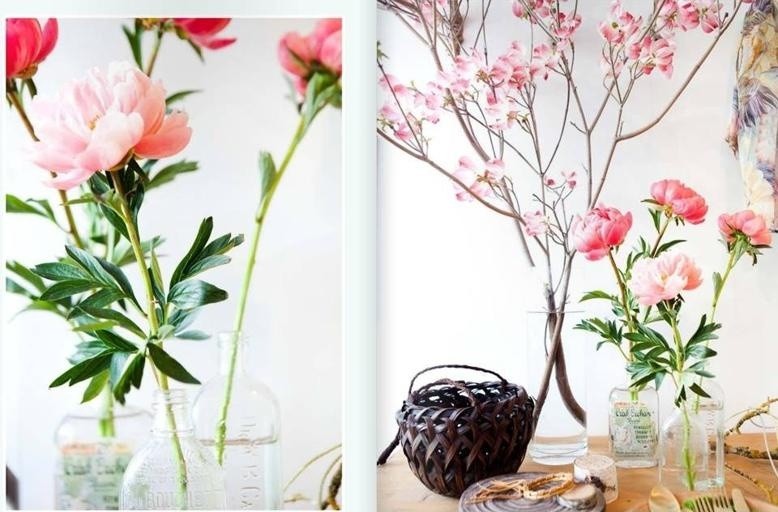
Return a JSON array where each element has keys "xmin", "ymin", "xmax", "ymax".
[{"xmin": 647, "ymin": 484, "xmax": 680, "ymax": 512}]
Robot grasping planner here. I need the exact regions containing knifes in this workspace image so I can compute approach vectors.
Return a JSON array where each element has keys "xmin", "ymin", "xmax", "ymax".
[
  {"xmin": 690, "ymin": 485, "xmax": 734, "ymax": 512},
  {"xmin": 730, "ymin": 487, "xmax": 751, "ymax": 512}
]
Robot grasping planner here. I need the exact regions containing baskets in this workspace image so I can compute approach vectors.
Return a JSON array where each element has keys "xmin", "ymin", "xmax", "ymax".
[{"xmin": 396, "ymin": 364, "xmax": 535, "ymax": 498}]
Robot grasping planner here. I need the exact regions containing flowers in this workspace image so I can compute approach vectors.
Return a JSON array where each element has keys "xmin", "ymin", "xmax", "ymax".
[{"xmin": 5, "ymin": 0, "xmax": 778, "ymax": 512}]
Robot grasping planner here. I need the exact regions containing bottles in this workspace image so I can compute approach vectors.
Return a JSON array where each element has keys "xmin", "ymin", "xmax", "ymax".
[{"xmin": 607, "ymin": 369, "xmax": 722, "ymax": 494}]
